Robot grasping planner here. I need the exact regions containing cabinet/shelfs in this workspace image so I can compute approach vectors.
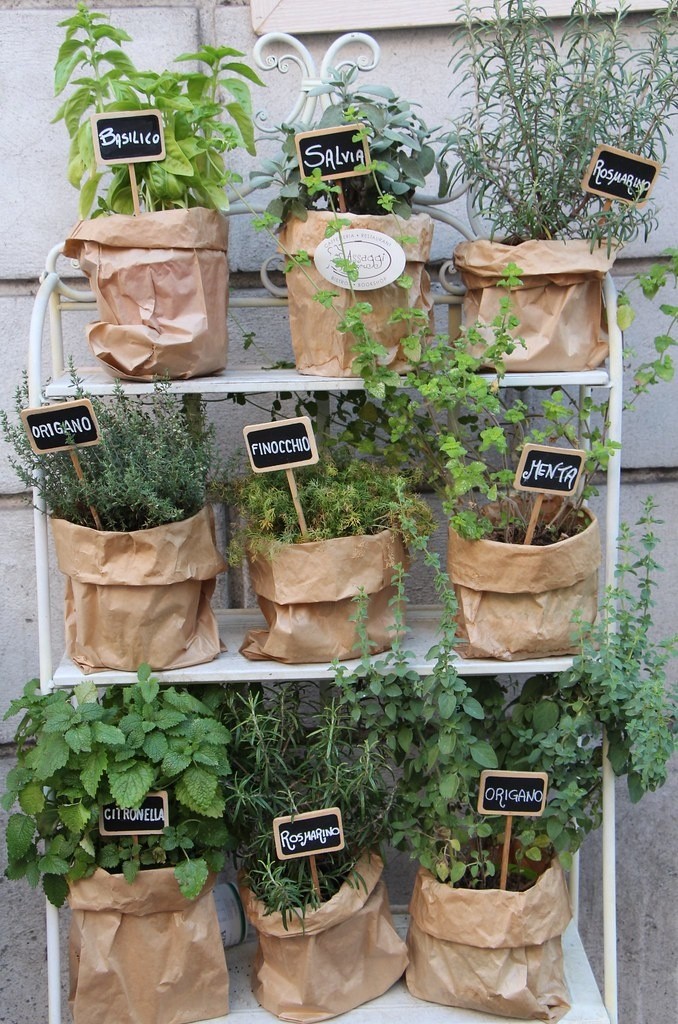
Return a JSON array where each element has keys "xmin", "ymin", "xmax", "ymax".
[{"xmin": 27, "ymin": 30, "xmax": 622, "ymax": 1024}]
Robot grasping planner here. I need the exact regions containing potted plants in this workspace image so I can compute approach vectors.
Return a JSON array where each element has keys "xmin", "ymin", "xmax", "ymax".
[{"xmin": 0, "ymin": 0, "xmax": 678, "ymax": 1024}]
